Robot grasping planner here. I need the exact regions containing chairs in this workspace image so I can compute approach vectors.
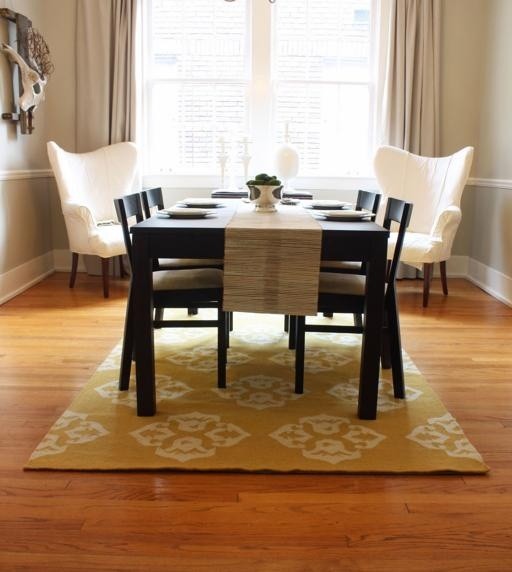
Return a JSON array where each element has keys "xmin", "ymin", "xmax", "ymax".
[
  {"xmin": 290, "ymin": 198, "xmax": 411, "ymax": 399},
  {"xmin": 376, "ymin": 146, "xmax": 473, "ymax": 303},
  {"xmin": 142, "ymin": 188, "xmax": 233, "ymax": 332},
  {"xmin": 112, "ymin": 193, "xmax": 229, "ymax": 388},
  {"xmin": 285, "ymin": 191, "xmax": 378, "ymax": 330},
  {"xmin": 46, "ymin": 140, "xmax": 149, "ymax": 298}
]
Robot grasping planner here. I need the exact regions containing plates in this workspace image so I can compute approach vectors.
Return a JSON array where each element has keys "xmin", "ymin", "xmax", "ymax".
[
  {"xmin": 181, "ymin": 201, "xmax": 222, "ymax": 208},
  {"xmin": 315, "ymin": 209, "xmax": 377, "ymax": 222},
  {"xmin": 307, "ymin": 201, "xmax": 349, "ymax": 209},
  {"xmin": 162, "ymin": 209, "xmax": 217, "ymax": 218}
]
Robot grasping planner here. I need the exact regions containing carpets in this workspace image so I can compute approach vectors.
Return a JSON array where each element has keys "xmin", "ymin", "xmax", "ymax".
[{"xmin": 24, "ymin": 308, "xmax": 491, "ymax": 478}]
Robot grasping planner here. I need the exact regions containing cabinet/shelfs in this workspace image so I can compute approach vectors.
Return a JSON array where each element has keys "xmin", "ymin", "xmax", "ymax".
[{"xmin": 210, "ymin": 190, "xmax": 315, "ymax": 200}]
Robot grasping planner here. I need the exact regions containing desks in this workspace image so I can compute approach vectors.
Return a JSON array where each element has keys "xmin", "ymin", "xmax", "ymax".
[{"xmin": 130, "ymin": 197, "xmax": 391, "ymax": 421}]
